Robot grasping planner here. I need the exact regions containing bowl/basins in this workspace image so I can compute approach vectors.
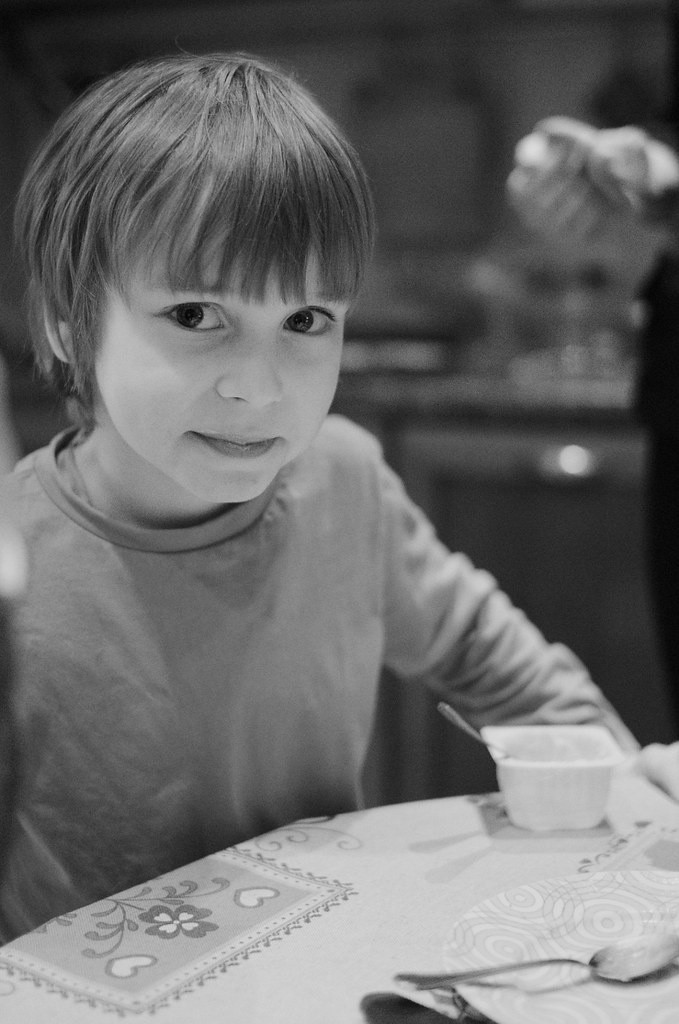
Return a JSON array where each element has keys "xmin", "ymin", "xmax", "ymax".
[{"xmin": 479, "ymin": 723, "xmax": 624, "ymax": 833}]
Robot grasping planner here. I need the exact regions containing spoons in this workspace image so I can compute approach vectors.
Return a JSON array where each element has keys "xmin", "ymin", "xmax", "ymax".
[{"xmin": 392, "ymin": 933, "xmax": 679, "ymax": 992}]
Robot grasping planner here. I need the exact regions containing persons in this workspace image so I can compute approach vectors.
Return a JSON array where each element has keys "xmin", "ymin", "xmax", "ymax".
[
  {"xmin": 0, "ymin": 48, "xmax": 679, "ymax": 950},
  {"xmin": 502, "ymin": 0, "xmax": 679, "ymax": 741}
]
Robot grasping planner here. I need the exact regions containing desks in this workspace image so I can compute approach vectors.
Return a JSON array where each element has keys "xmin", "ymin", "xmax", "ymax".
[{"xmin": 0, "ymin": 777, "xmax": 679, "ymax": 1024}]
